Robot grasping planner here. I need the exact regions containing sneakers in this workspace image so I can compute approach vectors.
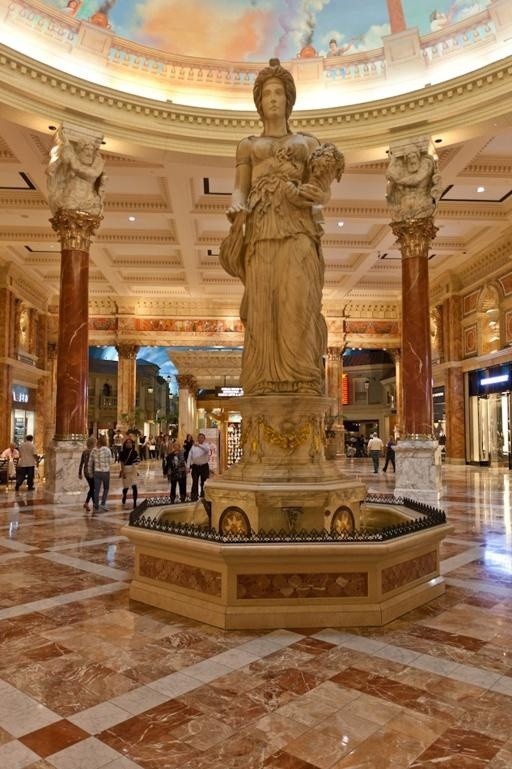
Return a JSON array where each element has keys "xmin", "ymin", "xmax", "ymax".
[
  {"xmin": 84, "ymin": 503, "xmax": 109, "ymax": 512},
  {"xmin": 15, "ymin": 487, "xmax": 35, "ymax": 491},
  {"xmin": 121, "ymin": 497, "xmax": 127, "ymax": 504}
]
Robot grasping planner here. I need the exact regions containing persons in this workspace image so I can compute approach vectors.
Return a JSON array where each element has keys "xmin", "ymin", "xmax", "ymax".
[
  {"xmin": 224, "ymin": 58, "xmax": 335, "ymax": 397},
  {"xmin": 384, "ymin": 146, "xmax": 434, "ymax": 211},
  {"xmin": 15, "ymin": 434, "xmax": 40, "ymax": 491},
  {"xmin": 367, "ymin": 473, "xmax": 395, "ymax": 495},
  {"xmin": 428, "ymin": 4, "xmax": 458, "ymax": 30},
  {"xmin": 45, "ymin": 140, "xmax": 106, "ymax": 214},
  {"xmin": 1, "ymin": 443, "xmax": 20, "ymax": 460},
  {"xmin": 367, "ymin": 431, "xmax": 384, "ymax": 473},
  {"xmin": 16, "ymin": 491, "xmax": 34, "ymax": 536},
  {"xmin": 380, "ymin": 434, "xmax": 395, "ymax": 472},
  {"xmin": 345, "ymin": 431, "xmax": 373, "ymax": 458},
  {"xmin": 326, "ymin": 38, "xmax": 354, "ymax": 57},
  {"xmin": 76, "ymin": 426, "xmax": 211, "ymax": 517}
]
